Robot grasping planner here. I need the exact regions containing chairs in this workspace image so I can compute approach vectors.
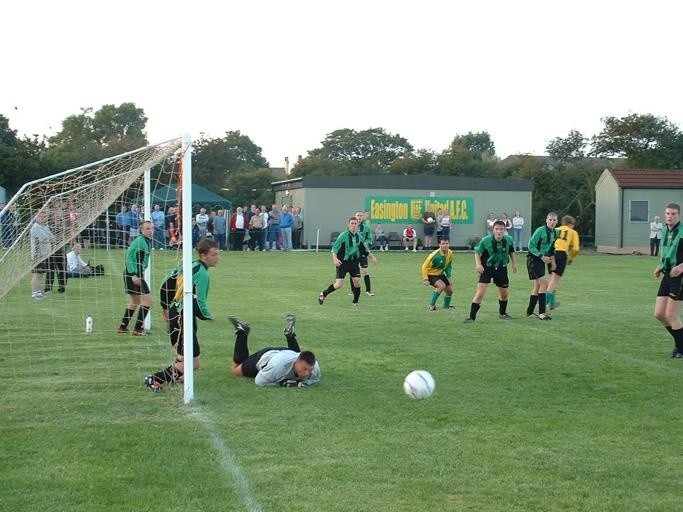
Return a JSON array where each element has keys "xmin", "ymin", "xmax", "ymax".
[
  {"xmin": 370, "ymin": 230, "xmax": 388, "ymax": 250},
  {"xmin": 388, "ymin": 232, "xmax": 401, "ymax": 248},
  {"xmin": 328, "ymin": 231, "xmax": 340, "ymax": 249}
]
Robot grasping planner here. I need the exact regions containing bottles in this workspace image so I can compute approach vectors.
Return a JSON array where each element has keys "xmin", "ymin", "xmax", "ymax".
[{"xmin": 85, "ymin": 314, "xmax": 93, "ymax": 332}]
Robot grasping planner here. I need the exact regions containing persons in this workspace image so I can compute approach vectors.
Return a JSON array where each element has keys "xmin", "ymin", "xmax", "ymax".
[
  {"xmin": 512, "ymin": 210, "xmax": 524, "ymax": 252},
  {"xmin": 525, "ymin": 212, "xmax": 559, "ymax": 322},
  {"xmin": 43, "ymin": 210, "xmax": 69, "ymax": 293},
  {"xmin": 402, "ymin": 204, "xmax": 455, "ymax": 311},
  {"xmin": 0, "ymin": 202, "xmax": 304, "ymax": 252},
  {"xmin": 486, "ymin": 212, "xmax": 497, "ymax": 235},
  {"xmin": 499, "ymin": 213, "xmax": 512, "ymax": 235},
  {"xmin": 67, "ymin": 244, "xmax": 105, "ymax": 278},
  {"xmin": 653, "ymin": 202, "xmax": 682, "ymax": 360},
  {"xmin": 143, "ymin": 240, "xmax": 220, "ymax": 393},
  {"xmin": 227, "ymin": 313, "xmax": 321, "ymax": 388},
  {"xmin": 649, "ymin": 215, "xmax": 663, "ymax": 257},
  {"xmin": 29, "ymin": 212, "xmax": 55, "ymax": 298},
  {"xmin": 463, "ymin": 220, "xmax": 517, "ymax": 323},
  {"xmin": 546, "ymin": 215, "xmax": 580, "ymax": 310},
  {"xmin": 318, "ymin": 210, "xmax": 390, "ymax": 308},
  {"xmin": 116, "ymin": 220, "xmax": 154, "ymax": 337}
]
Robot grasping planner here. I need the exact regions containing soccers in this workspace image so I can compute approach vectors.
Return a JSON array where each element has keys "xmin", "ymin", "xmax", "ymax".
[{"xmin": 403, "ymin": 370, "xmax": 436, "ymax": 402}]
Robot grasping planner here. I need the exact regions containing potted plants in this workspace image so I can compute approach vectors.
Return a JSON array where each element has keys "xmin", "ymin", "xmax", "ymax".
[
  {"xmin": 464, "ymin": 236, "xmax": 481, "ymax": 249},
  {"xmin": 416, "ymin": 238, "xmax": 424, "ymax": 250}
]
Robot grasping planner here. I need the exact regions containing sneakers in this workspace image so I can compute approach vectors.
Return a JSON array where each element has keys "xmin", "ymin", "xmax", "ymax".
[
  {"xmin": 527, "ymin": 313, "xmax": 551, "ymax": 320},
  {"xmin": 145, "ymin": 375, "xmax": 161, "ymax": 393},
  {"xmin": 498, "ymin": 313, "xmax": 512, "ymax": 320},
  {"xmin": 465, "ymin": 318, "xmax": 475, "ymax": 323},
  {"xmin": 428, "ymin": 304, "xmax": 436, "ymax": 311},
  {"xmin": 117, "ymin": 325, "xmax": 130, "ymax": 332},
  {"xmin": 133, "ymin": 328, "xmax": 152, "ymax": 336},
  {"xmin": 319, "ymin": 292, "xmax": 326, "ymax": 305},
  {"xmin": 351, "ymin": 302, "xmax": 359, "ymax": 306},
  {"xmin": 283, "ymin": 314, "xmax": 295, "ymax": 336},
  {"xmin": 229, "ymin": 316, "xmax": 250, "ymax": 333},
  {"xmin": 366, "ymin": 292, "xmax": 374, "ymax": 296}
]
